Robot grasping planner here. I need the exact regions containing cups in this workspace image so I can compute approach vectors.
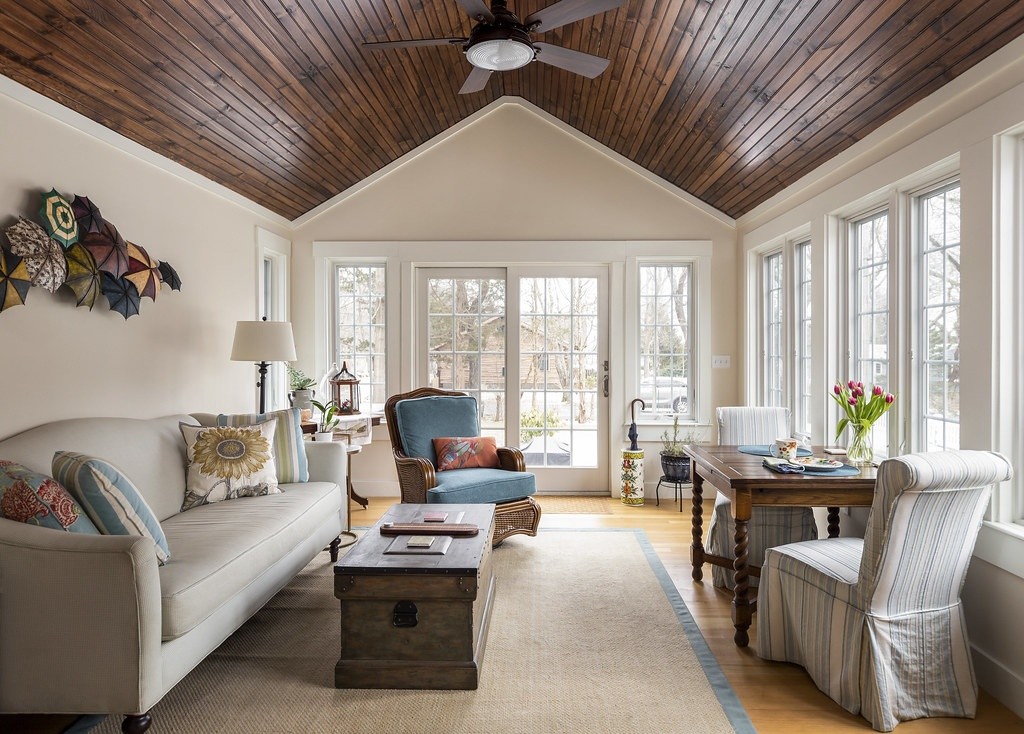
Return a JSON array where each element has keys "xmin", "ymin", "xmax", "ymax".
[{"xmin": 769, "ymin": 437, "xmax": 798, "ymax": 461}]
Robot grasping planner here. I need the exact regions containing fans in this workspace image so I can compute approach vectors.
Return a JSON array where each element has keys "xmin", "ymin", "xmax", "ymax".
[{"xmin": 362, "ymin": 0, "xmax": 630, "ymax": 95}]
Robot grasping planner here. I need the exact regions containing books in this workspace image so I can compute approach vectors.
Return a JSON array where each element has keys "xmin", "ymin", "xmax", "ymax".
[
  {"xmin": 424, "ymin": 512, "xmax": 448, "ymax": 521},
  {"xmin": 407, "ymin": 536, "xmax": 435, "ymax": 546}
]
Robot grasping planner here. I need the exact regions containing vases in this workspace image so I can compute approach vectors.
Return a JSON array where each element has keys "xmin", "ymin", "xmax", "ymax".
[{"xmin": 846, "ymin": 423, "xmax": 874, "ymax": 462}]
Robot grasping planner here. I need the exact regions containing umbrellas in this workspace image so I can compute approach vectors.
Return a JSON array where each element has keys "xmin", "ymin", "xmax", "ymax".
[
  {"xmin": 38, "ymin": 187, "xmax": 79, "ymax": 250},
  {"xmin": 0, "ymin": 249, "xmax": 32, "ymax": 312},
  {"xmin": 5, "ymin": 215, "xmax": 67, "ymax": 294},
  {"xmin": 158, "ymin": 260, "xmax": 182, "ymax": 292},
  {"xmin": 99, "ymin": 273, "xmax": 142, "ymax": 320},
  {"xmin": 63, "ymin": 242, "xmax": 101, "ymax": 312},
  {"xmin": 71, "ymin": 194, "xmax": 107, "ymax": 235},
  {"xmin": 80, "ymin": 219, "xmax": 130, "ymax": 280},
  {"xmin": 628, "ymin": 399, "xmax": 645, "ymax": 450},
  {"xmin": 122, "ymin": 241, "xmax": 163, "ymax": 302}
]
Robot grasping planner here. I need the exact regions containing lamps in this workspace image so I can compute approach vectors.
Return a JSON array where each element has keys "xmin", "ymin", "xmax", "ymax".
[
  {"xmin": 230, "ymin": 316, "xmax": 297, "ymax": 415},
  {"xmin": 464, "ymin": 23, "xmax": 535, "ymax": 71},
  {"xmin": 330, "ymin": 361, "xmax": 361, "ymax": 415}
]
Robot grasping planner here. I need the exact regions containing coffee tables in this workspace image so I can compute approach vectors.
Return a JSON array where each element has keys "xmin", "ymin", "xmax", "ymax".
[{"xmin": 333, "ymin": 504, "xmax": 497, "ymax": 691}]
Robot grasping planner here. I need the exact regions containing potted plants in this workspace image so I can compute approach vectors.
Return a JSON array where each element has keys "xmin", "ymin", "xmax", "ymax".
[
  {"xmin": 659, "ymin": 415, "xmax": 711, "ymax": 480},
  {"xmin": 284, "ymin": 361, "xmax": 340, "ymax": 443}
]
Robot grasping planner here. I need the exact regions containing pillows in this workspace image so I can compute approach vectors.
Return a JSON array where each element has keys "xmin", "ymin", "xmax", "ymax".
[
  {"xmin": 178, "ymin": 420, "xmax": 284, "ymax": 512},
  {"xmin": 52, "ymin": 451, "xmax": 171, "ymax": 567},
  {"xmin": 217, "ymin": 407, "xmax": 310, "ymax": 483},
  {"xmin": 433, "ymin": 437, "xmax": 503, "ymax": 471},
  {"xmin": 0, "ymin": 459, "xmax": 103, "ymax": 535}
]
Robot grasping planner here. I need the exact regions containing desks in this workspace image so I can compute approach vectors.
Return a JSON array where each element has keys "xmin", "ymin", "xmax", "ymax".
[
  {"xmin": 316, "ymin": 414, "xmax": 372, "ymax": 444},
  {"xmin": 684, "ymin": 445, "xmax": 885, "ymax": 648}
]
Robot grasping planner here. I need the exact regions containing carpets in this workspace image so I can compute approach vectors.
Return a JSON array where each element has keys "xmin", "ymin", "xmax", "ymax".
[{"xmin": 62, "ymin": 528, "xmax": 758, "ymax": 734}]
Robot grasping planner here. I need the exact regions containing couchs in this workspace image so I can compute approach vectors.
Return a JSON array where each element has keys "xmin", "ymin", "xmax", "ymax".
[{"xmin": 0, "ymin": 413, "xmax": 348, "ymax": 734}]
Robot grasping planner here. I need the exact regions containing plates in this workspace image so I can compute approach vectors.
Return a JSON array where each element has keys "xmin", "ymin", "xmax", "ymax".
[{"xmin": 789, "ymin": 456, "xmax": 844, "ymax": 472}]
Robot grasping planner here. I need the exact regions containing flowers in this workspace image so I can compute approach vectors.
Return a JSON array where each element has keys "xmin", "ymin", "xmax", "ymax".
[{"xmin": 828, "ymin": 379, "xmax": 899, "ymax": 461}]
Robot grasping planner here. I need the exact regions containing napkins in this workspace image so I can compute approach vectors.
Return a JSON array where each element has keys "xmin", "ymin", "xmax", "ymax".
[
  {"xmin": 763, "ymin": 457, "xmax": 805, "ymax": 475},
  {"xmin": 823, "ymin": 448, "xmax": 846, "ymax": 455}
]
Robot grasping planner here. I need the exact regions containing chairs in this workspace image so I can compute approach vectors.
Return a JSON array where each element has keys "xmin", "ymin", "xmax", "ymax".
[
  {"xmin": 705, "ymin": 406, "xmax": 818, "ymax": 592},
  {"xmin": 385, "ymin": 387, "xmax": 541, "ymax": 549},
  {"xmin": 758, "ymin": 450, "xmax": 1015, "ymax": 731}
]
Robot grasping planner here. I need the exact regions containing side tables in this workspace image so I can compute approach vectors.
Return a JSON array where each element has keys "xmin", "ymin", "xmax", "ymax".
[{"xmin": 344, "ymin": 444, "xmax": 368, "ymax": 510}]
